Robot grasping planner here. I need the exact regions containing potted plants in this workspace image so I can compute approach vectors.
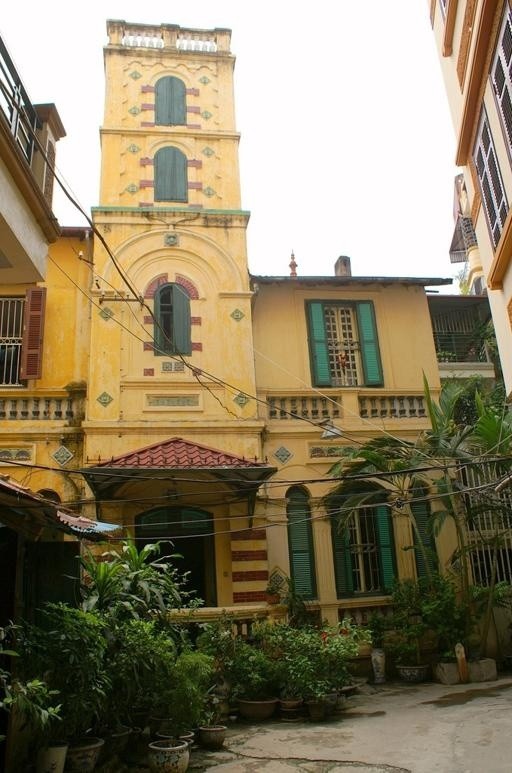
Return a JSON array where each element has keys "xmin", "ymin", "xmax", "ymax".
[{"xmin": 0, "ymin": 526, "xmax": 442, "ymax": 773}]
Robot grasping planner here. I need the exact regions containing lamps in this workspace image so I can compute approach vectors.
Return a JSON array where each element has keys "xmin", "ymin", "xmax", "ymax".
[{"xmin": 312, "ymin": 417, "xmax": 342, "ymax": 440}]
